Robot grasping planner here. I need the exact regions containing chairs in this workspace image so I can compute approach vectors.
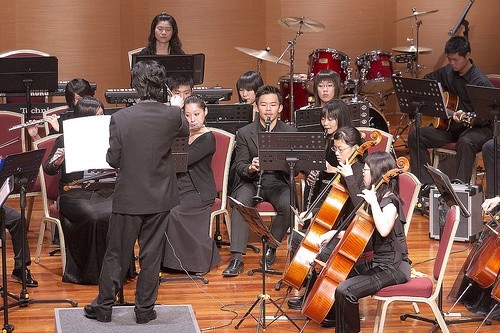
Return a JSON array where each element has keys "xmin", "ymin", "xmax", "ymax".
[
  {"xmin": 33, "ymin": 133, "xmax": 67, "ymax": 276},
  {"xmin": 397, "ymin": 172, "xmax": 421, "ymax": 238},
  {"xmin": 43, "ymin": 106, "xmax": 72, "ymax": 136},
  {"xmin": 0, "ymin": 111, "xmax": 25, "ymax": 157},
  {"xmin": 370, "ymin": 205, "xmax": 461, "ymax": 333},
  {"xmin": 206, "ymin": 127, "xmax": 236, "ymax": 241},
  {"xmin": 352, "ymin": 127, "xmax": 394, "ymax": 156},
  {"xmin": 0, "ymin": 49, "xmax": 53, "ymax": 123},
  {"xmin": 253, "ymin": 201, "xmax": 299, "ymax": 236},
  {"xmin": 432, "ymin": 74, "xmax": 500, "ymax": 187}
]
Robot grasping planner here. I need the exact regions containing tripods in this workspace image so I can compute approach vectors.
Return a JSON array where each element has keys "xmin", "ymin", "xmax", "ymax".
[
  {"xmin": 0, "ymin": 149, "xmax": 79, "ymax": 312},
  {"xmin": 400, "ymin": 163, "xmax": 491, "ymax": 333},
  {"xmin": 226, "ymin": 196, "xmax": 304, "ymax": 333},
  {"xmin": 391, "ymin": 74, "xmax": 449, "ymax": 215},
  {"xmin": 259, "ymin": 130, "xmax": 326, "ymax": 290},
  {"xmin": 200, "ymin": 104, "xmax": 262, "ymax": 254}
]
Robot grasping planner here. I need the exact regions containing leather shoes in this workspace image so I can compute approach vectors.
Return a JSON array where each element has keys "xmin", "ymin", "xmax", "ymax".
[
  {"xmin": 137, "ymin": 311, "xmax": 157, "ymax": 323},
  {"xmin": 259, "ymin": 246, "xmax": 276, "ymax": 266},
  {"xmin": 84, "ymin": 304, "xmax": 111, "ymax": 322},
  {"xmin": 287, "ymin": 297, "xmax": 302, "ymax": 309},
  {"xmin": 11, "ymin": 267, "xmax": 38, "ymax": 286},
  {"xmin": 321, "ymin": 316, "xmax": 336, "ymax": 327},
  {"xmin": 223, "ymin": 259, "xmax": 244, "ymax": 275}
]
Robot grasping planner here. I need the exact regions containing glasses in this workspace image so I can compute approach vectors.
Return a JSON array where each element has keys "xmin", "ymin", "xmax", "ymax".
[
  {"xmin": 317, "ymin": 83, "xmax": 336, "ymax": 88},
  {"xmin": 361, "ymin": 167, "xmax": 370, "ymax": 173},
  {"xmin": 330, "ymin": 146, "xmax": 352, "ymax": 153}
]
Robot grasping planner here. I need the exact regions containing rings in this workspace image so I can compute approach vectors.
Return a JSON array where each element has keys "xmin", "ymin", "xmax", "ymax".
[{"xmin": 340, "ymin": 167, "xmax": 342, "ymax": 171}]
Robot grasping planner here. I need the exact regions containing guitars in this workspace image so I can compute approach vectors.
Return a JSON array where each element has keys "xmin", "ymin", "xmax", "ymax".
[
  {"xmin": 416, "ymin": 88, "xmax": 477, "ymax": 130},
  {"xmin": 342, "ymin": 94, "xmax": 391, "ymax": 135}
]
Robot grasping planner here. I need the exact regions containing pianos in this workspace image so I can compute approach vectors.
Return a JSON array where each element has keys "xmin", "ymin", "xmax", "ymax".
[
  {"xmin": 102, "ymin": 86, "xmax": 235, "ymax": 105},
  {"xmin": 0, "ymin": 80, "xmax": 98, "ymax": 98}
]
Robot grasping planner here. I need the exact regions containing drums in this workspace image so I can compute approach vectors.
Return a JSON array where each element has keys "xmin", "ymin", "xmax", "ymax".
[
  {"xmin": 277, "ymin": 72, "xmax": 311, "ymax": 126},
  {"xmin": 303, "ymin": 47, "xmax": 352, "ymax": 96},
  {"xmin": 354, "ymin": 47, "xmax": 399, "ymax": 96}
]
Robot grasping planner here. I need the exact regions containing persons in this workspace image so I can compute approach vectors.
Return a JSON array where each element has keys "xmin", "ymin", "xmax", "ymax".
[
  {"xmin": 84, "ymin": 59, "xmax": 190, "ymax": 324},
  {"xmin": 0, "ymin": 155, "xmax": 38, "ymax": 293},
  {"xmin": 408, "ymin": 35, "xmax": 498, "ymax": 198},
  {"xmin": 317, "ymin": 151, "xmax": 413, "ymax": 333},
  {"xmin": 130, "ymin": 13, "xmax": 186, "ymax": 89},
  {"xmin": 234, "ymin": 70, "xmax": 360, "ymax": 211},
  {"xmin": 482, "ymin": 110, "xmax": 500, "ymax": 320},
  {"xmin": 288, "ymin": 126, "xmax": 366, "ymax": 310},
  {"xmin": 26, "ymin": 74, "xmax": 196, "ymax": 141},
  {"xmin": 221, "ymin": 85, "xmax": 300, "ymax": 276},
  {"xmin": 44, "ymin": 94, "xmax": 138, "ymax": 285},
  {"xmin": 162, "ymin": 96, "xmax": 222, "ymax": 276}
]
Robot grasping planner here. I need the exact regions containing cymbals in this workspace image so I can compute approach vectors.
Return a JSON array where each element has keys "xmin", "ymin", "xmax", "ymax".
[
  {"xmin": 391, "ymin": 10, "xmax": 439, "ymax": 24},
  {"xmin": 233, "ymin": 46, "xmax": 291, "ymax": 66},
  {"xmin": 277, "ymin": 15, "xmax": 327, "ymax": 33},
  {"xmin": 389, "ymin": 45, "xmax": 433, "ymax": 55}
]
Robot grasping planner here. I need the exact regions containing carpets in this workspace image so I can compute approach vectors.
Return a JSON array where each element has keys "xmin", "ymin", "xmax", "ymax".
[{"xmin": 54, "ymin": 304, "xmax": 200, "ymax": 333}]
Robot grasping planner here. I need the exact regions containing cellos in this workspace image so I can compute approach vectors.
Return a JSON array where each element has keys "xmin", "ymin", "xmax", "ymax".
[
  {"xmin": 279, "ymin": 128, "xmax": 386, "ymax": 292},
  {"xmin": 447, "ymin": 218, "xmax": 500, "ymax": 333},
  {"xmin": 300, "ymin": 156, "xmax": 412, "ymax": 325}
]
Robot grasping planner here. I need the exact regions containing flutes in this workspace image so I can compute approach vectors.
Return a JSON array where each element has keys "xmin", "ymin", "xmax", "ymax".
[
  {"xmin": 189, "ymin": 124, "xmax": 206, "ymax": 129},
  {"xmin": 48, "ymin": 152, "xmax": 65, "ymax": 165},
  {"xmin": 252, "ymin": 116, "xmax": 274, "ymax": 204},
  {"xmin": 8, "ymin": 116, "xmax": 61, "ymax": 131}
]
